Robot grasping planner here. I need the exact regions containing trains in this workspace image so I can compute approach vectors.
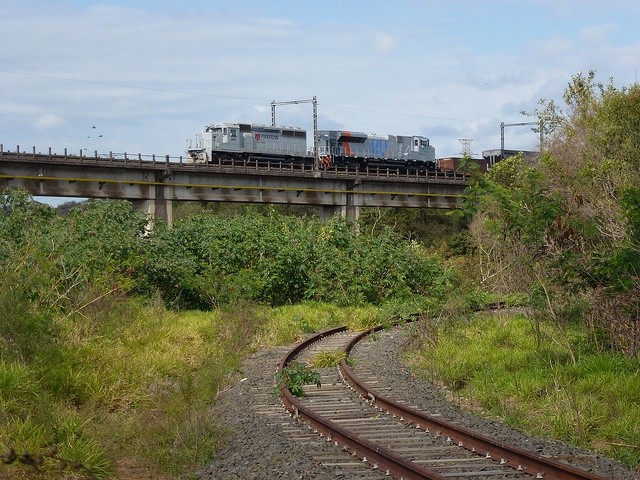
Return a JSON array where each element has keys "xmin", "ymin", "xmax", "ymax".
[{"xmin": 185, "ymin": 123, "xmax": 542, "ymax": 179}]
